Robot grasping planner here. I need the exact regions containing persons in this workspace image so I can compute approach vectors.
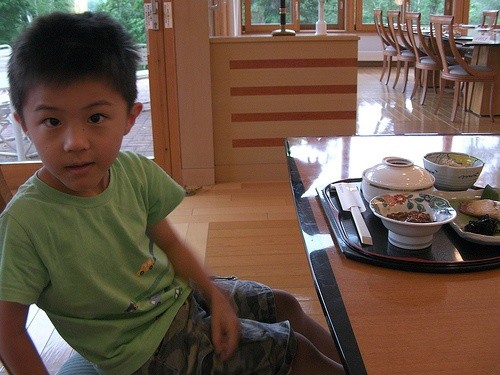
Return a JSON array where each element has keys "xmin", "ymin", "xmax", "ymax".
[{"xmin": 0, "ymin": 11, "xmax": 346, "ymax": 375}]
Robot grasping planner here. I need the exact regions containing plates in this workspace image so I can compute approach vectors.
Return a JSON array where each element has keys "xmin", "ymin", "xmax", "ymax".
[{"xmin": 439, "ymin": 189, "xmax": 500, "ymax": 245}]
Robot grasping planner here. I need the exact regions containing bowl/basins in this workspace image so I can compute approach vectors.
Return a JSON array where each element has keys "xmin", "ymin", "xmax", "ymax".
[
  {"xmin": 424, "ymin": 151, "xmax": 486, "ymax": 191},
  {"xmin": 371, "ymin": 193, "xmax": 457, "ymax": 250},
  {"xmin": 360, "ymin": 157, "xmax": 436, "ymax": 205}
]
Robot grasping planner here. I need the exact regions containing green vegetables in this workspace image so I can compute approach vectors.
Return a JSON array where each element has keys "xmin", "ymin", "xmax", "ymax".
[{"xmin": 463, "ymin": 213, "xmax": 500, "ymax": 236}]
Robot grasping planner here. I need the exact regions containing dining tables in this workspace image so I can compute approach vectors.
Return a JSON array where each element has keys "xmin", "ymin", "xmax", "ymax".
[{"xmin": 284, "ymin": 133, "xmax": 500, "ymax": 375}]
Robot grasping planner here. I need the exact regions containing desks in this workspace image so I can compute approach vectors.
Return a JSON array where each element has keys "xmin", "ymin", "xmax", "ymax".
[{"xmin": 379, "ymin": 22, "xmax": 500, "ymax": 116}]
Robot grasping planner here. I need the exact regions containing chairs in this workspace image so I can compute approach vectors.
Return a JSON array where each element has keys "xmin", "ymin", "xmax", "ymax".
[{"xmin": 372, "ymin": 9, "xmax": 499, "ymax": 121}]
